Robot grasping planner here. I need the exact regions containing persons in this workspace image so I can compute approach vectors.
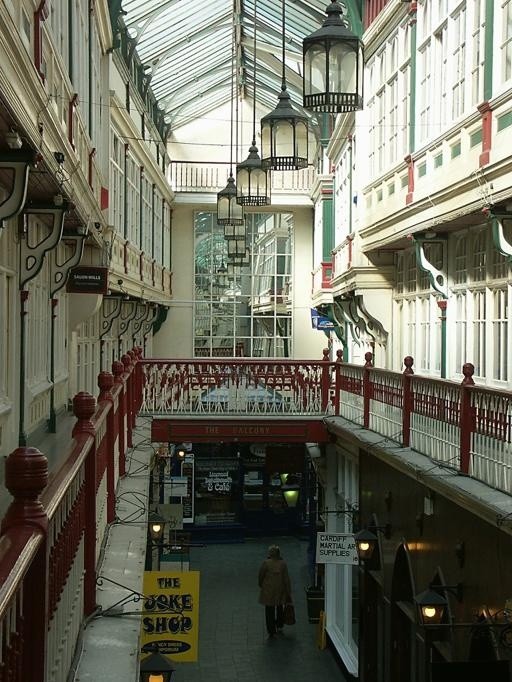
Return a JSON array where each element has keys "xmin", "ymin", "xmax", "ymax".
[{"xmin": 258, "ymin": 543, "xmax": 291, "ymax": 640}]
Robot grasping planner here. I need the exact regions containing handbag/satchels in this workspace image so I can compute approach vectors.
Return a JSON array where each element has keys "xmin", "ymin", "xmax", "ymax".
[{"xmin": 283, "ymin": 600, "xmax": 296, "ymax": 625}]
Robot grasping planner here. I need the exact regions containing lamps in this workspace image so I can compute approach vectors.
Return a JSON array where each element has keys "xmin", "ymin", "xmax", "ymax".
[
  {"xmin": 233, "ymin": 248, "xmax": 250, "ymax": 267},
  {"xmin": 226, "ymin": 240, "xmax": 248, "ymax": 257},
  {"xmin": 296, "ymin": 2, "xmax": 364, "ymax": 115},
  {"xmin": 354, "ymin": 523, "xmax": 390, "ymax": 563},
  {"xmin": 215, "ymin": 178, "xmax": 246, "ymax": 227},
  {"xmin": 140, "ymin": 645, "xmax": 179, "ymax": 681},
  {"xmin": 221, "ymin": 215, "xmax": 246, "ymax": 240},
  {"xmin": 234, "ymin": 148, "xmax": 271, "ymax": 208},
  {"xmin": 275, "ymin": 463, "xmax": 358, "ymax": 526},
  {"xmin": 260, "ymin": 99, "xmax": 309, "ymax": 172},
  {"xmin": 412, "ymin": 580, "xmax": 463, "ymax": 631}
]
